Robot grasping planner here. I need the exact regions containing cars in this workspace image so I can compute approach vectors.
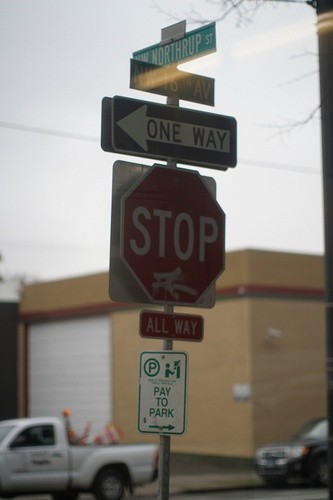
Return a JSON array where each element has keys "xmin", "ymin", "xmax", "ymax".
[{"xmin": 252, "ymin": 417, "xmax": 333, "ymax": 488}]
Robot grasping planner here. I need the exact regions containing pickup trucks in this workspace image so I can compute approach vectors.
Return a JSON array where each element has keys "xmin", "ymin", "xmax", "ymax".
[{"xmin": 0, "ymin": 414, "xmax": 160, "ymax": 500}]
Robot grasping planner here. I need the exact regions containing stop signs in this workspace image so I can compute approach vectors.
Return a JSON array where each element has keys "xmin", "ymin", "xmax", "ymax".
[{"xmin": 119, "ymin": 162, "xmax": 227, "ymax": 307}]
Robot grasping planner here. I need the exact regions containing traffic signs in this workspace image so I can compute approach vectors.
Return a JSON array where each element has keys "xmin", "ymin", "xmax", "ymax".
[{"xmin": 111, "ymin": 95, "xmax": 238, "ymax": 169}]
